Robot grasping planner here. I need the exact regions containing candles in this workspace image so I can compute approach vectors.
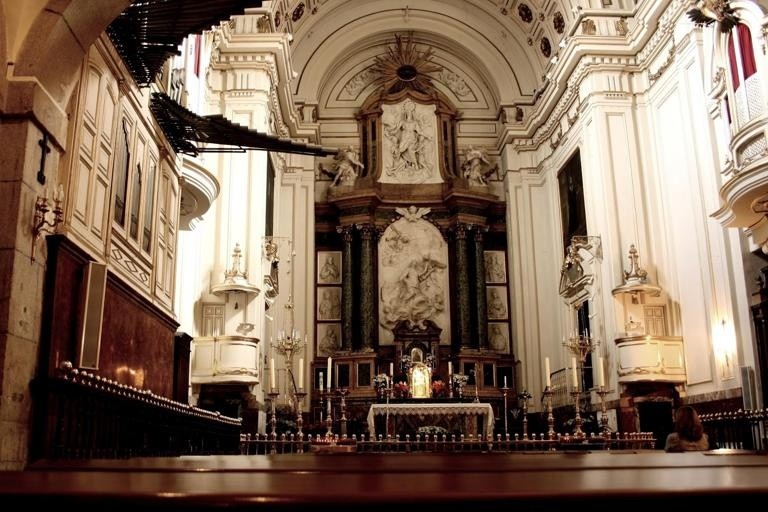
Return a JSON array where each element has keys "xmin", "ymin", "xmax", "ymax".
[
  {"xmin": 598, "ymin": 357, "xmax": 605, "ymax": 387},
  {"xmin": 298, "ymin": 359, "xmax": 304, "ymax": 388},
  {"xmin": 448, "ymin": 362, "xmax": 453, "ymax": 375},
  {"xmin": 571, "ymin": 357, "xmax": 579, "ymax": 388},
  {"xmin": 545, "ymin": 357, "xmax": 550, "ymax": 386},
  {"xmin": 327, "ymin": 358, "xmax": 332, "ymax": 388},
  {"xmin": 270, "ymin": 359, "xmax": 276, "ymax": 388},
  {"xmin": 390, "ymin": 362, "xmax": 394, "ymax": 377}
]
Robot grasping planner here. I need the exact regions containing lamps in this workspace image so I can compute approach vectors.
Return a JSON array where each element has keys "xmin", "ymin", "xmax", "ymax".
[{"xmin": 30, "ymin": 183, "xmax": 64, "ymax": 265}]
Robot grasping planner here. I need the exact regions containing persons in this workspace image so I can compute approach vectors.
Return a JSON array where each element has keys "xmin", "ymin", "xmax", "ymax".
[
  {"xmin": 406, "ymin": 205, "xmax": 419, "ymax": 222},
  {"xmin": 380, "ymin": 229, "xmax": 446, "ymax": 331},
  {"xmin": 387, "ymin": 109, "xmax": 433, "ymax": 172},
  {"xmin": 318, "ymin": 254, "xmax": 343, "ymax": 357},
  {"xmin": 484, "ymin": 253, "xmax": 508, "ymax": 352},
  {"xmin": 330, "ymin": 143, "xmax": 366, "ymax": 188},
  {"xmin": 665, "ymin": 406, "xmax": 710, "ymax": 451},
  {"xmin": 464, "ymin": 143, "xmax": 491, "ymax": 187}
]
